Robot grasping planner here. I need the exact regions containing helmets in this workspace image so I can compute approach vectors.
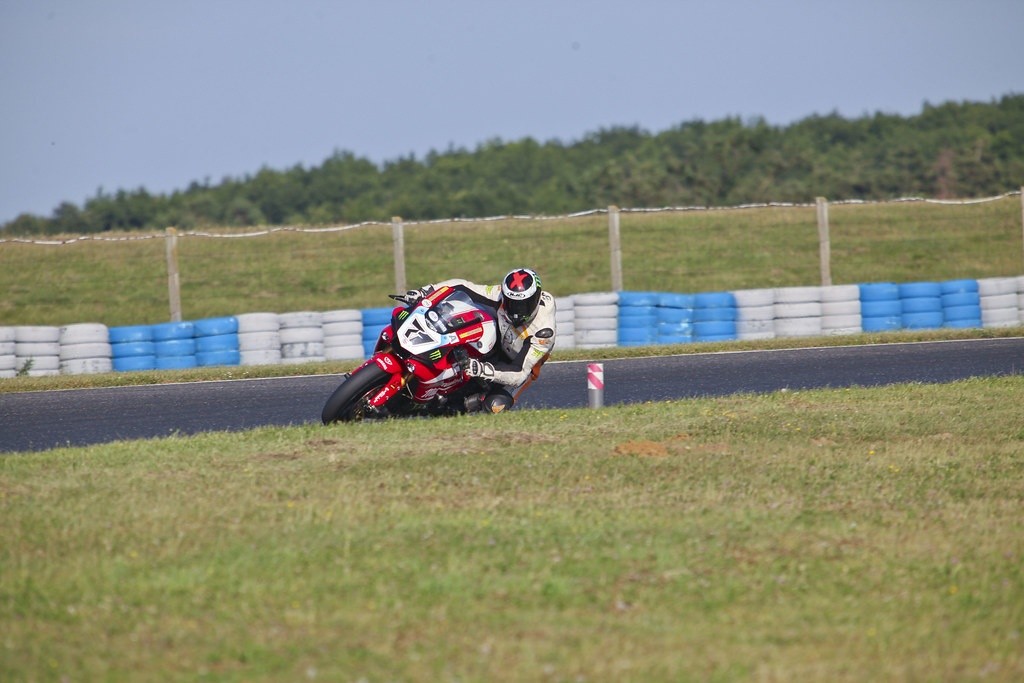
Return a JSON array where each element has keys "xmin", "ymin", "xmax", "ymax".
[{"xmin": 501, "ymin": 268, "xmax": 542, "ymax": 326}]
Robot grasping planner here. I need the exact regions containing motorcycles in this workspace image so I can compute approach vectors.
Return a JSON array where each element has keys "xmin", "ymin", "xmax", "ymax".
[{"xmin": 319, "ymin": 285, "xmax": 513, "ymax": 425}]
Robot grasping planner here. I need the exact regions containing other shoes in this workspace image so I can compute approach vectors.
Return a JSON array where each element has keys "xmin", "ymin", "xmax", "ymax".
[{"xmin": 464, "ymin": 392, "xmax": 486, "ymax": 411}]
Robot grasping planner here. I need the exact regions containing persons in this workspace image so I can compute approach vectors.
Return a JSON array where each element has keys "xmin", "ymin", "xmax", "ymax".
[{"xmin": 403, "ymin": 268, "xmax": 556, "ymax": 414}]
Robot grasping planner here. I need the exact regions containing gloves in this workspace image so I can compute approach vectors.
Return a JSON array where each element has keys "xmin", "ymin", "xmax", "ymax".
[
  {"xmin": 403, "ymin": 284, "xmax": 435, "ymax": 304},
  {"xmin": 457, "ymin": 358, "xmax": 494, "ymax": 383}
]
